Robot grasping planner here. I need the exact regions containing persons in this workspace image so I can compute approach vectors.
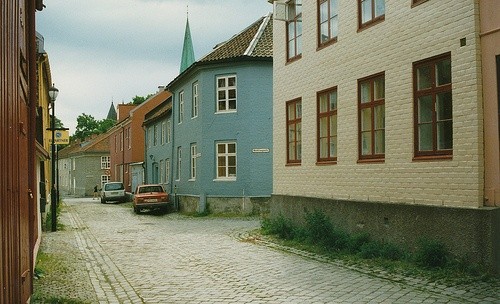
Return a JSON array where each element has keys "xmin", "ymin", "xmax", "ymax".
[{"xmin": 93, "ymin": 184, "xmax": 99, "ymax": 200}]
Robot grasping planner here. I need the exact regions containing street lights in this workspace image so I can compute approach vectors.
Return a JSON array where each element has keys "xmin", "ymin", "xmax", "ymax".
[{"xmin": 48, "ymin": 82, "xmax": 60, "ymax": 232}]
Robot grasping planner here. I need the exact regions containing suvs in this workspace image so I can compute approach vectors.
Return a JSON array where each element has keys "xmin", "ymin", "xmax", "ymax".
[{"xmin": 99, "ymin": 182, "xmax": 126, "ymax": 203}]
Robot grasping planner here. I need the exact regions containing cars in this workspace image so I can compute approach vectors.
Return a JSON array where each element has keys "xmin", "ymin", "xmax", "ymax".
[{"xmin": 130, "ymin": 183, "xmax": 170, "ymax": 214}]
